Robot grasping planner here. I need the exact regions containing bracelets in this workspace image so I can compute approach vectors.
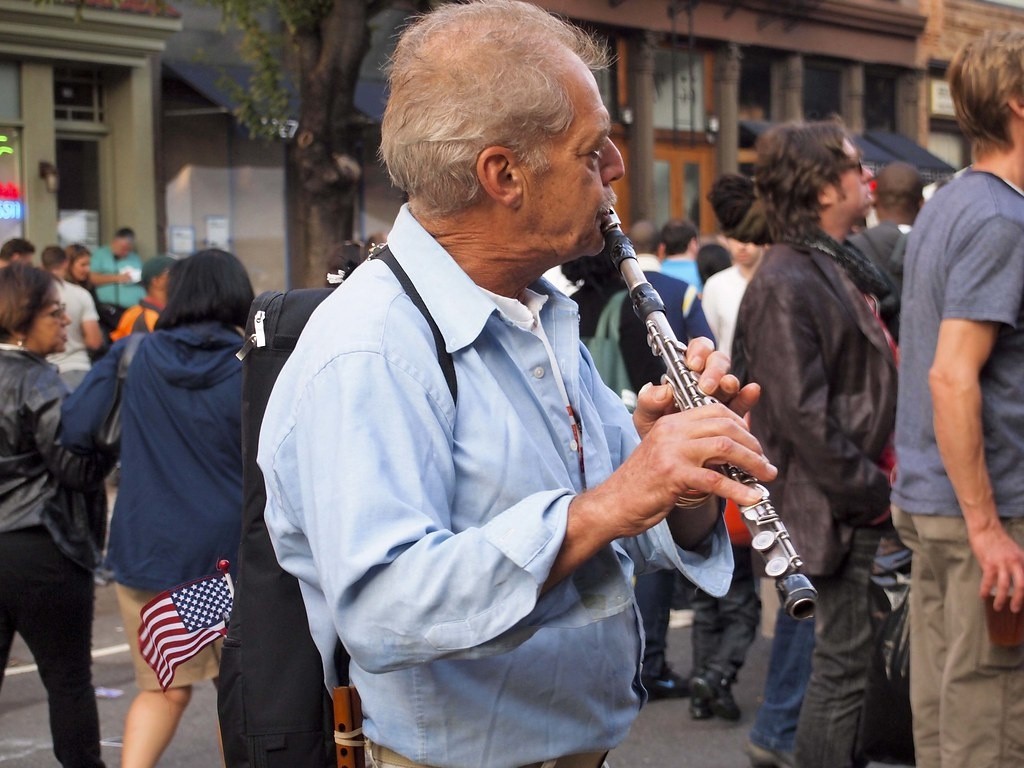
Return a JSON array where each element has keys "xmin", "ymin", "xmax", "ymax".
[{"xmin": 671, "ymin": 486, "xmax": 712, "ymax": 509}]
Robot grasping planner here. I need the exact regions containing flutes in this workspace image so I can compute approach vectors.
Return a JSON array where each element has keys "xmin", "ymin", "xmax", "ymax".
[{"xmin": 600, "ymin": 206, "xmax": 818, "ymax": 620}]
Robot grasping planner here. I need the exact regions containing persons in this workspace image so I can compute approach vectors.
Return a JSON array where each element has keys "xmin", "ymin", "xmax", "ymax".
[
  {"xmin": 563, "ymin": 236, "xmax": 717, "ymax": 701},
  {"xmin": 631, "ymin": 175, "xmax": 773, "ymax": 378},
  {"xmin": 255, "ymin": 1, "xmax": 780, "ymax": 767},
  {"xmin": 1, "ymin": 225, "xmax": 254, "ymax": 767},
  {"xmin": 742, "ymin": 575, "xmax": 815, "ymax": 766},
  {"xmin": 853, "ymin": 22, "xmax": 1024, "ymax": 768},
  {"xmin": 731, "ymin": 117, "xmax": 914, "ymax": 766},
  {"xmin": 687, "ymin": 497, "xmax": 763, "ymax": 723}
]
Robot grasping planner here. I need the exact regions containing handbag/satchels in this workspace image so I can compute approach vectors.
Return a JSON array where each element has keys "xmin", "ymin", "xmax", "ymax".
[
  {"xmin": 873, "ymin": 587, "xmax": 911, "ymax": 684},
  {"xmin": 95, "ymin": 331, "xmax": 146, "ymax": 449}
]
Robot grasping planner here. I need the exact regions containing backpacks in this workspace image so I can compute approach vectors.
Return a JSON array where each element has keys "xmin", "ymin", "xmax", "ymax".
[{"xmin": 217, "ymin": 243, "xmax": 455, "ymax": 768}]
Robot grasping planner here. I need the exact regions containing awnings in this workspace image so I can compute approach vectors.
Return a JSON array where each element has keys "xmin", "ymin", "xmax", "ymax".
[
  {"xmin": 863, "ymin": 128, "xmax": 957, "ymax": 185},
  {"xmin": 737, "ymin": 118, "xmax": 905, "ymax": 181},
  {"xmin": 353, "ymin": 78, "xmax": 386, "ymax": 123},
  {"xmin": 159, "ymin": 56, "xmax": 303, "ymax": 140}
]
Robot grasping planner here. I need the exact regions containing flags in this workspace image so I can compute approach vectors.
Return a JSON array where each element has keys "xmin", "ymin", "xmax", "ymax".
[{"xmin": 136, "ymin": 561, "xmax": 238, "ymax": 694}]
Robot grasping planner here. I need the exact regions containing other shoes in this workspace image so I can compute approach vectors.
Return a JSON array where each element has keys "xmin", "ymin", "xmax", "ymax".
[
  {"xmin": 689, "ymin": 670, "xmax": 740, "ymax": 720},
  {"xmin": 632, "ymin": 662, "xmax": 689, "ymax": 696},
  {"xmin": 692, "ymin": 695, "xmax": 711, "ymax": 718},
  {"xmin": 739, "ymin": 736, "xmax": 795, "ymax": 768}
]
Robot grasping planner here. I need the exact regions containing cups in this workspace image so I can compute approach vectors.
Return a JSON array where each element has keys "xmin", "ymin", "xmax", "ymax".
[{"xmin": 981, "ymin": 578, "xmax": 1024, "ymax": 646}]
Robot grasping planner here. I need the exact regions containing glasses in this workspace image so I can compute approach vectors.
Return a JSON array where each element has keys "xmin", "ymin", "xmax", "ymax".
[{"xmin": 837, "ymin": 158, "xmax": 863, "ymax": 173}]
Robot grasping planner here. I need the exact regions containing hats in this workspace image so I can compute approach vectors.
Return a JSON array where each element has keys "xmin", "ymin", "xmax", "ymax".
[{"xmin": 136, "ymin": 256, "xmax": 176, "ymax": 285}]
